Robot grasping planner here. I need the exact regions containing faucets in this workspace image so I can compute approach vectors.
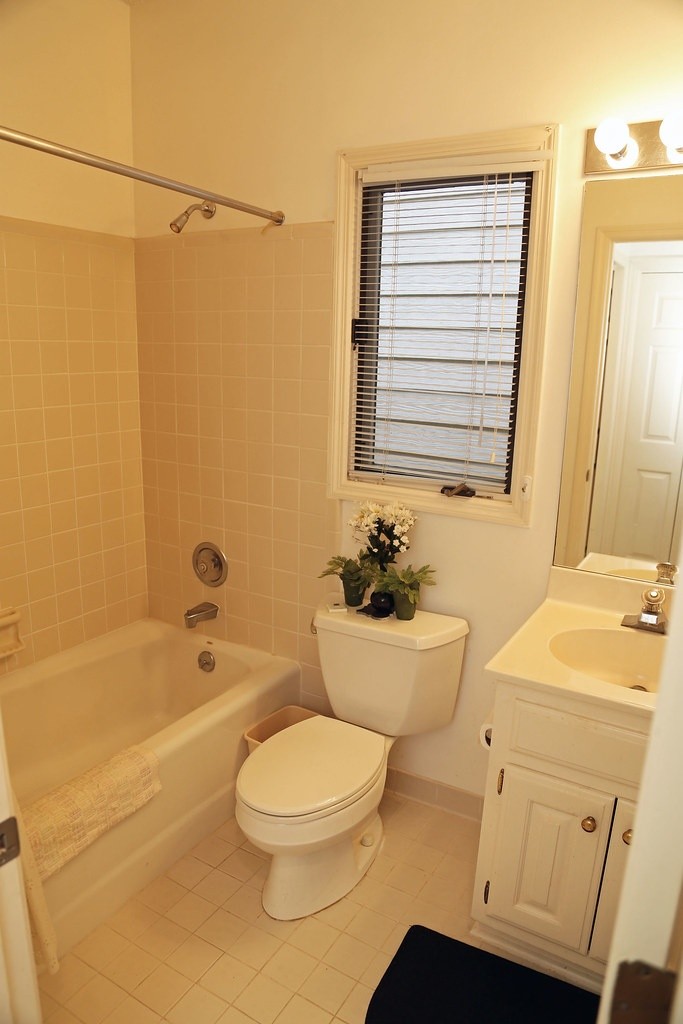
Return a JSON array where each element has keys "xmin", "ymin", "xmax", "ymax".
[
  {"xmin": 654, "ymin": 561, "xmax": 679, "ymax": 587},
  {"xmin": 637, "ymin": 589, "xmax": 665, "ymax": 627},
  {"xmin": 180, "ymin": 599, "xmax": 213, "ymax": 631}
]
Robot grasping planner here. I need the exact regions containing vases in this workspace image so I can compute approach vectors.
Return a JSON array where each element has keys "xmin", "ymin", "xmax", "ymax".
[{"xmin": 370, "ymin": 564, "xmax": 394, "ymax": 612}]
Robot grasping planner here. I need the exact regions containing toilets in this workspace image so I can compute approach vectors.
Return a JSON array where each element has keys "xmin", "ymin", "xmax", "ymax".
[{"xmin": 226, "ymin": 599, "xmax": 472, "ymax": 923}]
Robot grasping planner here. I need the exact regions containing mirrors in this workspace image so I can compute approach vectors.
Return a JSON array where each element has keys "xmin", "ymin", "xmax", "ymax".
[{"xmin": 552, "ymin": 166, "xmax": 683, "ymax": 591}]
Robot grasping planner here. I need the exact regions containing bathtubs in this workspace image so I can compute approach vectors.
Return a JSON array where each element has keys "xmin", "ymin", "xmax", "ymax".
[{"xmin": 0, "ymin": 609, "xmax": 303, "ymax": 965}]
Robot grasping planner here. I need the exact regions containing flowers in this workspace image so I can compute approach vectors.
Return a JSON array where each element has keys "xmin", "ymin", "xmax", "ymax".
[{"xmin": 347, "ymin": 499, "xmax": 419, "ymax": 563}]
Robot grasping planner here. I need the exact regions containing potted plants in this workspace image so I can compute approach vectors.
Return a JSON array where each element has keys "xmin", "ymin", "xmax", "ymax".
[
  {"xmin": 319, "ymin": 548, "xmax": 378, "ymax": 606},
  {"xmin": 373, "ymin": 564, "xmax": 438, "ymax": 620}
]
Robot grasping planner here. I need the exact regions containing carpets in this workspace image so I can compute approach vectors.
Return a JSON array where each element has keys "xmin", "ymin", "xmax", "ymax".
[{"xmin": 365, "ymin": 925, "xmax": 601, "ymax": 1024}]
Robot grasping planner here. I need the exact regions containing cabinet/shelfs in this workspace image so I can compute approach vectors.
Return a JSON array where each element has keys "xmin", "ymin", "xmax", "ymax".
[{"xmin": 468, "ymin": 683, "xmax": 653, "ymax": 994}]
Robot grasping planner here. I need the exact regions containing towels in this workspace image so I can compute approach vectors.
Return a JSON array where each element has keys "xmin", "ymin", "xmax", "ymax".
[{"xmin": 21, "ymin": 739, "xmax": 163, "ymax": 883}]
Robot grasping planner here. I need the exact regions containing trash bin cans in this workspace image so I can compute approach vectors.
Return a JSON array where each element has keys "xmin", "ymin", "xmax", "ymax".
[{"xmin": 242, "ymin": 703, "xmax": 320, "ymax": 756}]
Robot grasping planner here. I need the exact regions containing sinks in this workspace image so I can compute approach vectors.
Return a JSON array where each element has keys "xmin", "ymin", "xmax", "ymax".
[
  {"xmin": 546, "ymin": 624, "xmax": 670, "ymax": 695},
  {"xmin": 605, "ymin": 567, "xmax": 676, "ymax": 584}
]
